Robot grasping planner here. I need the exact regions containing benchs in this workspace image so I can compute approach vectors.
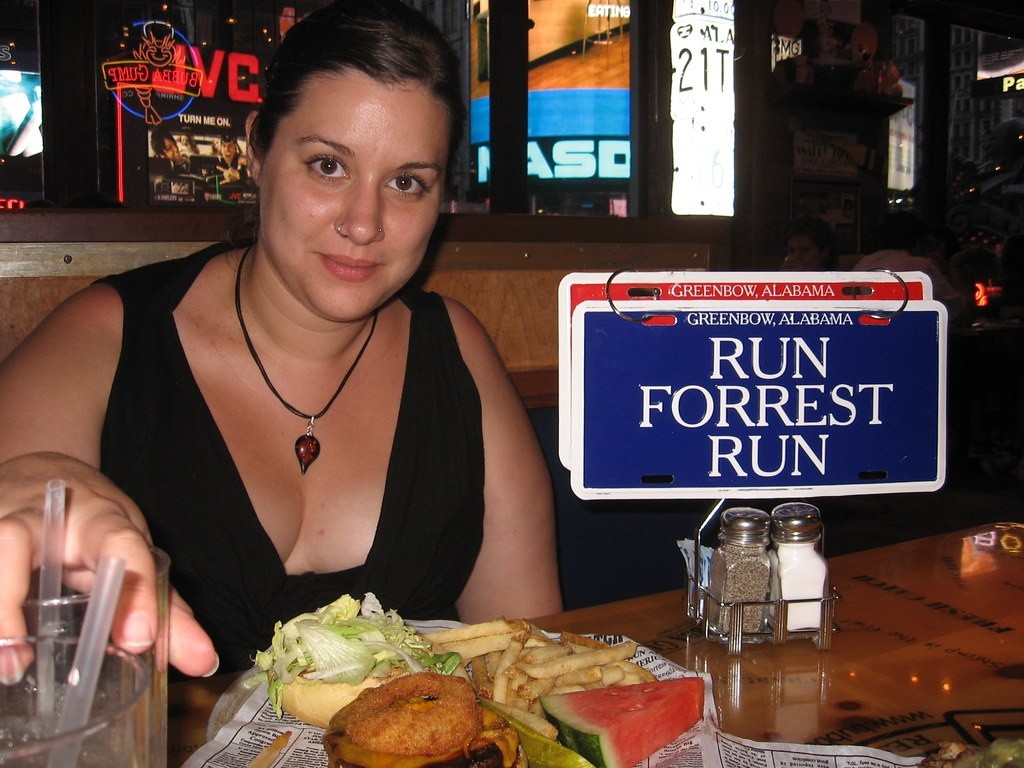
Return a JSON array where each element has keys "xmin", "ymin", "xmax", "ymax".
[{"xmin": 502, "ymin": 365, "xmax": 865, "ymax": 614}]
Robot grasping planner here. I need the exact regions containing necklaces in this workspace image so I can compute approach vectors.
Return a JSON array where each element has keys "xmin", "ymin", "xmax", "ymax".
[{"xmin": 235, "ymin": 243, "xmax": 378, "ymax": 476}]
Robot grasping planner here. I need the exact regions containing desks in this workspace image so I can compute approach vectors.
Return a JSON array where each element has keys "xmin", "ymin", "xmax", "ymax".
[{"xmin": 167, "ymin": 522, "xmax": 1024, "ymax": 768}]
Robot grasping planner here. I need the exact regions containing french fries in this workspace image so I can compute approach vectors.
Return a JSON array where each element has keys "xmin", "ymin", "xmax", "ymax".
[{"xmin": 413, "ymin": 615, "xmax": 658, "ymax": 740}]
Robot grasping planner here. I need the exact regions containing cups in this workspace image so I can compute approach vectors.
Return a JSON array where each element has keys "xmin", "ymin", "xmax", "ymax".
[{"xmin": 0, "ymin": 547, "xmax": 170, "ymax": 767}]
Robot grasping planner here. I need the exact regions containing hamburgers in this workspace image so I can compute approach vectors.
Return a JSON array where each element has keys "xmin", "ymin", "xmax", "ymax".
[{"xmin": 248, "ymin": 591, "xmax": 528, "ymax": 768}]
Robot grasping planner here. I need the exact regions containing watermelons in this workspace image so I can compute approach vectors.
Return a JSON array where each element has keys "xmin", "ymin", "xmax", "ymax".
[{"xmin": 539, "ymin": 676, "xmax": 704, "ymax": 768}]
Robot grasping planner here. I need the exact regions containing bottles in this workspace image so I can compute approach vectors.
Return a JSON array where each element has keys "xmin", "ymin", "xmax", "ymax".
[
  {"xmin": 770, "ymin": 503, "xmax": 827, "ymax": 633},
  {"xmin": 708, "ymin": 508, "xmax": 771, "ymax": 636}
]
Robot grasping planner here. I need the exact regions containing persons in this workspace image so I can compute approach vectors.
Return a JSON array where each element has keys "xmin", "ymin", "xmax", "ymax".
[
  {"xmin": 0, "ymin": 0, "xmax": 563, "ymax": 685},
  {"xmin": 150, "ymin": 128, "xmax": 248, "ymax": 185},
  {"xmin": 779, "ymin": 208, "xmax": 977, "ymax": 329}
]
